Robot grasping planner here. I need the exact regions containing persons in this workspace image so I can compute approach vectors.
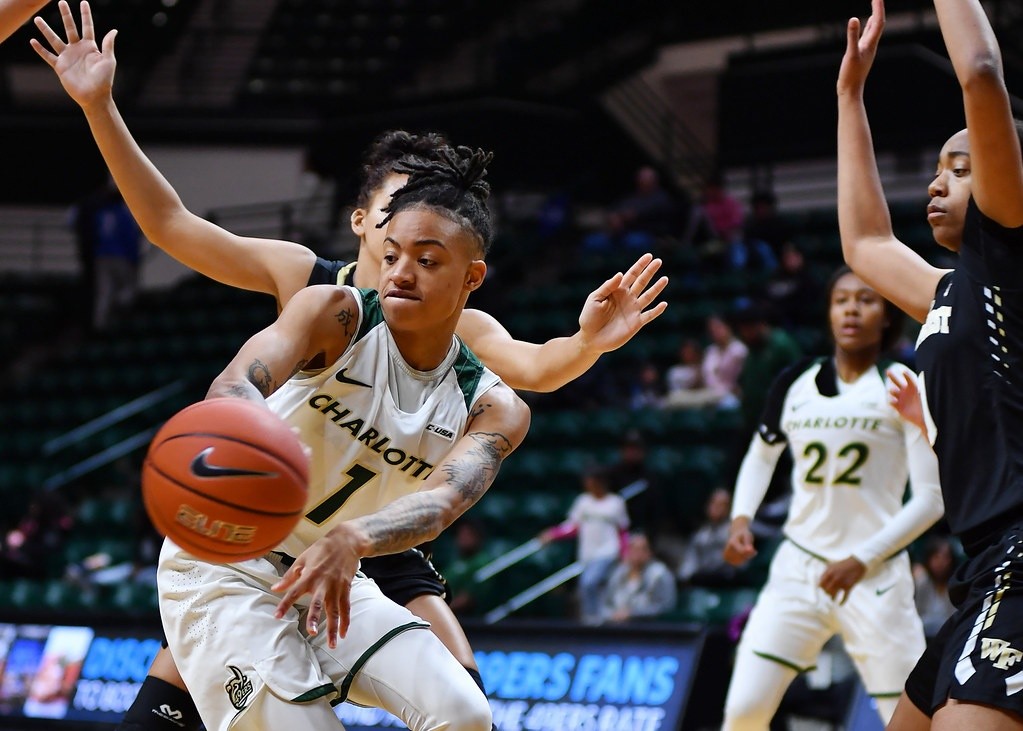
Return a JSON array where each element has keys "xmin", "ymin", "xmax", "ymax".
[
  {"xmin": 280, "ymin": 203, "xmax": 321, "ymax": 252},
  {"xmin": 440, "ymin": 524, "xmax": 495, "ymax": 613},
  {"xmin": 58, "ymin": 166, "xmax": 146, "ymax": 329},
  {"xmin": 835, "ymin": 0, "xmax": 1023, "ymax": 731},
  {"xmin": 914, "ymin": 538, "xmax": 960, "ymax": 639},
  {"xmin": 884, "ymin": 368, "xmax": 930, "ymax": 447},
  {"xmin": 155, "ymin": 144, "xmax": 530, "ymax": 731},
  {"xmin": 537, "ymin": 163, "xmax": 819, "ymax": 623},
  {"xmin": 723, "ymin": 265, "xmax": 945, "ymax": 730},
  {"xmin": 6, "ymin": 494, "xmax": 72, "ymax": 570},
  {"xmin": 27, "ymin": 0, "xmax": 669, "ymax": 731}
]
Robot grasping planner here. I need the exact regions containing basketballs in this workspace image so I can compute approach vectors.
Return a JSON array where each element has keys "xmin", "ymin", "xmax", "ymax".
[{"xmin": 141, "ymin": 396, "xmax": 310, "ymax": 564}]
